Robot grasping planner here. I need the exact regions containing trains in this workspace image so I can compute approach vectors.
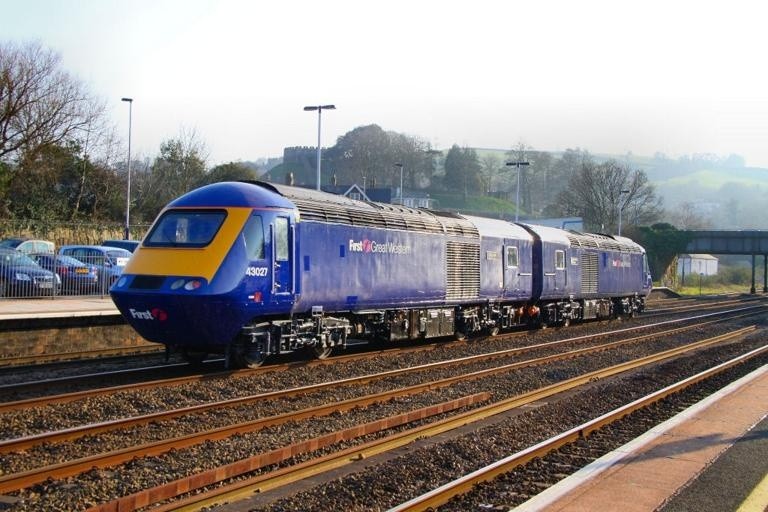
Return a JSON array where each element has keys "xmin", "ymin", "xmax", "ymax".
[{"xmin": 106, "ymin": 175, "xmax": 655, "ymax": 375}]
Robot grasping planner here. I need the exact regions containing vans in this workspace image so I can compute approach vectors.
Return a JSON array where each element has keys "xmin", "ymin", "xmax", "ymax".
[
  {"xmin": 57, "ymin": 241, "xmax": 135, "ymax": 294},
  {"xmin": 101, "ymin": 239, "xmax": 141, "ymax": 254},
  {"xmin": 0, "ymin": 237, "xmax": 56, "ymax": 256}
]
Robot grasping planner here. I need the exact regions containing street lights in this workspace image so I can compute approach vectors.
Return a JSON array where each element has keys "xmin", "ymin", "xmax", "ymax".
[
  {"xmin": 302, "ymin": 103, "xmax": 336, "ymax": 189},
  {"xmin": 119, "ymin": 97, "xmax": 133, "ymax": 239},
  {"xmin": 618, "ymin": 190, "xmax": 632, "ymax": 236},
  {"xmin": 394, "ymin": 162, "xmax": 402, "ymax": 203},
  {"xmin": 504, "ymin": 162, "xmax": 529, "ymax": 223}
]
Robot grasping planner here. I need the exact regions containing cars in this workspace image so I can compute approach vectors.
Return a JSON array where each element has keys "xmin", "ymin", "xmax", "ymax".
[
  {"xmin": 31, "ymin": 249, "xmax": 100, "ymax": 296},
  {"xmin": 0, "ymin": 245, "xmax": 63, "ymax": 297}
]
